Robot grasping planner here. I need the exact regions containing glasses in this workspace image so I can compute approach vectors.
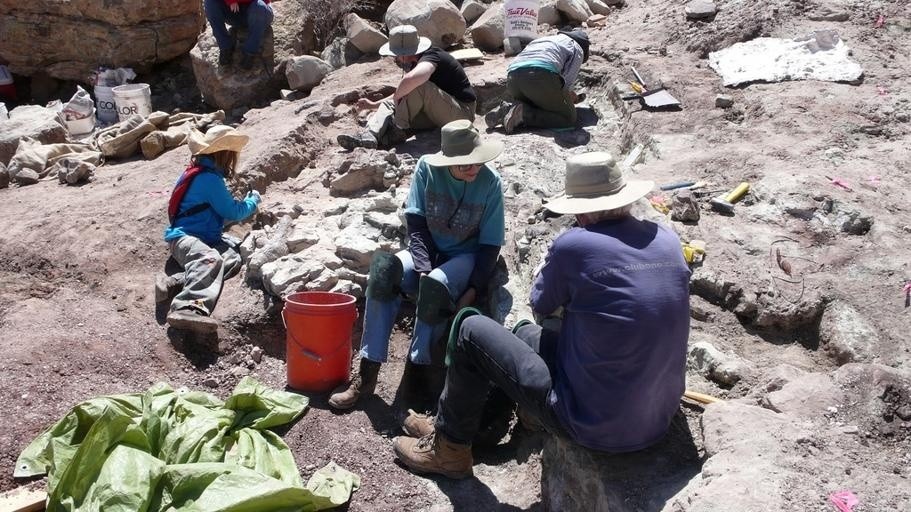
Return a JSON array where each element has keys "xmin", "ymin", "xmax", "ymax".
[{"xmin": 458, "ymin": 163, "xmax": 484, "ymax": 172}]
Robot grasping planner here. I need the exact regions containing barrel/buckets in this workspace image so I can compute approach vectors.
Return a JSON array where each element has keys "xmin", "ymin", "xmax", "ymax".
[
  {"xmin": 504, "ymin": 0, "xmax": 541, "ymax": 40},
  {"xmin": 279, "ymin": 290, "xmax": 361, "ymax": 396},
  {"xmin": 92, "ymin": 82, "xmax": 118, "ymax": 123},
  {"xmin": 112, "ymin": 82, "xmax": 154, "ymax": 122}
]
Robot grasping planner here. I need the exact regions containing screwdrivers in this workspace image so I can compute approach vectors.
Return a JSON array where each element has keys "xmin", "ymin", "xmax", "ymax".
[{"xmin": 626, "ymin": 79, "xmax": 643, "ymax": 92}]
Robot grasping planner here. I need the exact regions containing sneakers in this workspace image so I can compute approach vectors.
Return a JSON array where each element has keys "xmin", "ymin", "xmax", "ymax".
[
  {"xmin": 328, "ymin": 372, "xmax": 377, "ymax": 409},
  {"xmin": 166, "ymin": 309, "xmax": 218, "ymax": 335},
  {"xmin": 240, "ymin": 49, "xmax": 252, "ymax": 69},
  {"xmin": 379, "ymin": 125, "xmax": 406, "ymax": 146},
  {"xmin": 337, "ymin": 133, "xmax": 377, "ymax": 151},
  {"xmin": 392, "ymin": 414, "xmax": 473, "ymax": 479},
  {"xmin": 155, "ymin": 271, "xmax": 169, "ymax": 302},
  {"xmin": 485, "ymin": 104, "xmax": 510, "ymax": 128},
  {"xmin": 503, "ymin": 99, "xmax": 523, "ymax": 135},
  {"xmin": 219, "ymin": 42, "xmax": 232, "ymax": 64}
]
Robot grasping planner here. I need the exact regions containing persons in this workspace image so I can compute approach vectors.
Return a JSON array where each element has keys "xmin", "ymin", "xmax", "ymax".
[
  {"xmin": 393, "ymin": 150, "xmax": 694, "ymax": 482},
  {"xmin": 484, "ymin": 31, "xmax": 592, "ymax": 135},
  {"xmin": 327, "ymin": 119, "xmax": 505, "ymax": 427},
  {"xmin": 337, "ymin": 22, "xmax": 477, "ymax": 149},
  {"xmin": 155, "ymin": 125, "xmax": 264, "ymax": 336},
  {"xmin": 204, "ymin": 1, "xmax": 273, "ymax": 71}
]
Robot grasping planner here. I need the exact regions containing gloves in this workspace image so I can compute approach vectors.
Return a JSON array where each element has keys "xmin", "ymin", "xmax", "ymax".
[{"xmin": 246, "ymin": 189, "xmax": 262, "ymax": 205}]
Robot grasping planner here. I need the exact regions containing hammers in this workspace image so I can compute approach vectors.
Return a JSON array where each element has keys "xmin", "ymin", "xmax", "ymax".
[{"xmin": 710, "ymin": 182, "xmax": 749, "ymax": 213}]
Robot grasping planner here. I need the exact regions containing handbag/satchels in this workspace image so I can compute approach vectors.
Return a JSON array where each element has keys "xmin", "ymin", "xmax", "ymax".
[{"xmin": 168, "ymin": 164, "xmax": 222, "ymax": 225}]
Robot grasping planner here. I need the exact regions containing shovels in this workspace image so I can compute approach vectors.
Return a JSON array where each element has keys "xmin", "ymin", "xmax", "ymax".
[{"xmin": 623, "ymin": 87, "xmax": 682, "ymax": 110}]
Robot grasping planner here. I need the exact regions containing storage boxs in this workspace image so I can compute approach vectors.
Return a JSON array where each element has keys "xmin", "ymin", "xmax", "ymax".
[{"xmin": 63, "ymin": 107, "xmax": 96, "ymax": 134}]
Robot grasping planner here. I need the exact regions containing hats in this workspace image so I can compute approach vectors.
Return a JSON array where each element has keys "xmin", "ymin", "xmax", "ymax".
[
  {"xmin": 557, "ymin": 30, "xmax": 591, "ymax": 64},
  {"xmin": 424, "ymin": 119, "xmax": 504, "ymax": 167},
  {"xmin": 541, "ymin": 151, "xmax": 655, "ymax": 214},
  {"xmin": 187, "ymin": 125, "xmax": 249, "ymax": 156},
  {"xmin": 379, "ymin": 25, "xmax": 432, "ymax": 56}
]
